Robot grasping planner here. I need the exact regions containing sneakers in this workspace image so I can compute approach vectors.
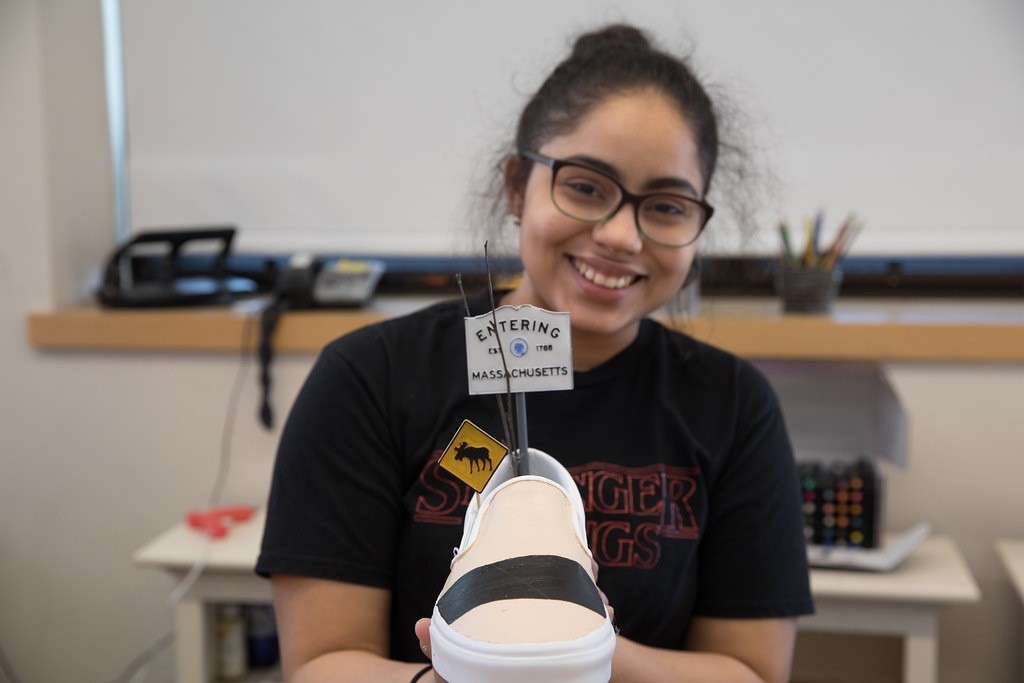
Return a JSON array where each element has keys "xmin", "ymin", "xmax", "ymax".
[{"xmin": 429, "ymin": 449, "xmax": 615, "ymax": 683}]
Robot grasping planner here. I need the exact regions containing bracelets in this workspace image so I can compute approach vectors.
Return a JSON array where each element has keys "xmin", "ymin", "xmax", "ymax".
[{"xmin": 410, "ymin": 666, "xmax": 436, "ymax": 681}]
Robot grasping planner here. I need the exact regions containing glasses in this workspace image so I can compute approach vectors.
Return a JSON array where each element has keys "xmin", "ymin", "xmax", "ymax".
[{"xmin": 521, "ymin": 150, "xmax": 714, "ymax": 248}]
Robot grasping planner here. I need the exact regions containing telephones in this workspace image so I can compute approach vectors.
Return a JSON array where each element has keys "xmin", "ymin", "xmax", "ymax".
[{"xmin": 281, "ymin": 252, "xmax": 388, "ymax": 307}]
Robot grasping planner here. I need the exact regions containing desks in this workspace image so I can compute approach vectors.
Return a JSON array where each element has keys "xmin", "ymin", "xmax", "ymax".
[{"xmin": 133, "ymin": 511, "xmax": 981, "ymax": 683}]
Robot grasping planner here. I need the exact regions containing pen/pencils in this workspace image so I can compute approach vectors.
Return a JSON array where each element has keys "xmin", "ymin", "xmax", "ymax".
[{"xmin": 777, "ymin": 211, "xmax": 865, "ymax": 270}]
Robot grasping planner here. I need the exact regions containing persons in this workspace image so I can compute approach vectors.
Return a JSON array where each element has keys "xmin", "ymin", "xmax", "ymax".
[{"xmin": 252, "ymin": 21, "xmax": 816, "ymax": 682}]
